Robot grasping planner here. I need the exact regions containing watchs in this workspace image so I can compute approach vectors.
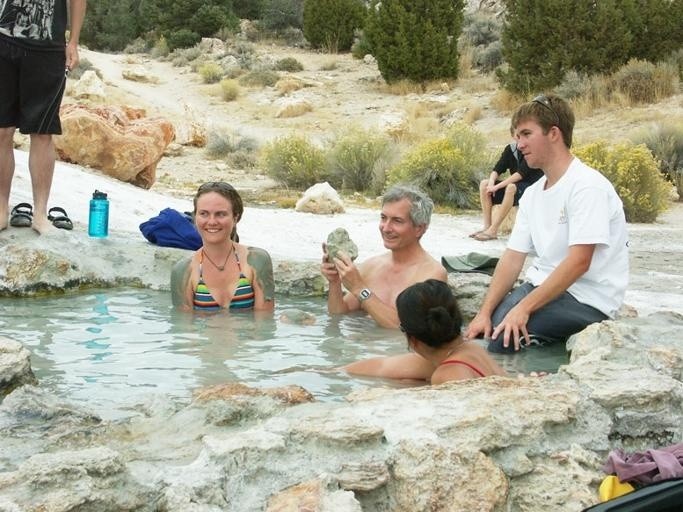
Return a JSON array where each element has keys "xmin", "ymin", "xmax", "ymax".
[{"xmin": 357, "ymin": 289, "xmax": 372, "ymax": 303}]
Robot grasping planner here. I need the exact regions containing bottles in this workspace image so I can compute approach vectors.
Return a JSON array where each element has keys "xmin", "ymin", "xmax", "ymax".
[{"xmin": 88, "ymin": 190, "xmax": 108, "ymax": 237}]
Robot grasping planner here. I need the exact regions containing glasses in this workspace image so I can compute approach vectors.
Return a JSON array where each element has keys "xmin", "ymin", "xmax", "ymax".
[
  {"xmin": 198, "ymin": 182, "xmax": 234, "ymax": 191},
  {"xmin": 533, "ymin": 95, "xmax": 559, "ymax": 126}
]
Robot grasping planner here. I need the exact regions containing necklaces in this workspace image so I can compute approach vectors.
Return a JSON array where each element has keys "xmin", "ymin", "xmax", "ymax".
[{"xmin": 203, "ymin": 245, "xmax": 233, "ymax": 271}]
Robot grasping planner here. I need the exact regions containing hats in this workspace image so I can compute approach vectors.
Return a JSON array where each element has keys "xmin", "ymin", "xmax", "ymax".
[{"xmin": 442, "ymin": 253, "xmax": 499, "ymax": 275}]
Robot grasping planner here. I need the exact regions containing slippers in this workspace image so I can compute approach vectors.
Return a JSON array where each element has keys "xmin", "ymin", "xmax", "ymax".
[{"xmin": 472, "ymin": 231, "xmax": 497, "ymax": 240}]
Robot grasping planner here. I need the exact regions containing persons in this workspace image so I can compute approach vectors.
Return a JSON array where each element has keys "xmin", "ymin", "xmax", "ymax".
[
  {"xmin": 0, "ymin": 0, "xmax": 87, "ymax": 242},
  {"xmin": 170, "ymin": 181, "xmax": 275, "ymax": 316},
  {"xmin": 468, "ymin": 124, "xmax": 544, "ymax": 239},
  {"xmin": 320, "ymin": 180, "xmax": 447, "ymax": 329},
  {"xmin": 336, "ymin": 278, "xmax": 509, "ymax": 386},
  {"xmin": 461, "ymin": 95, "xmax": 631, "ymax": 355}
]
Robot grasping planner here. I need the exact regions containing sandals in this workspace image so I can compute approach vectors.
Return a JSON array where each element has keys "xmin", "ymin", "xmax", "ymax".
[
  {"xmin": 47, "ymin": 207, "xmax": 72, "ymax": 229},
  {"xmin": 10, "ymin": 203, "xmax": 33, "ymax": 226}
]
